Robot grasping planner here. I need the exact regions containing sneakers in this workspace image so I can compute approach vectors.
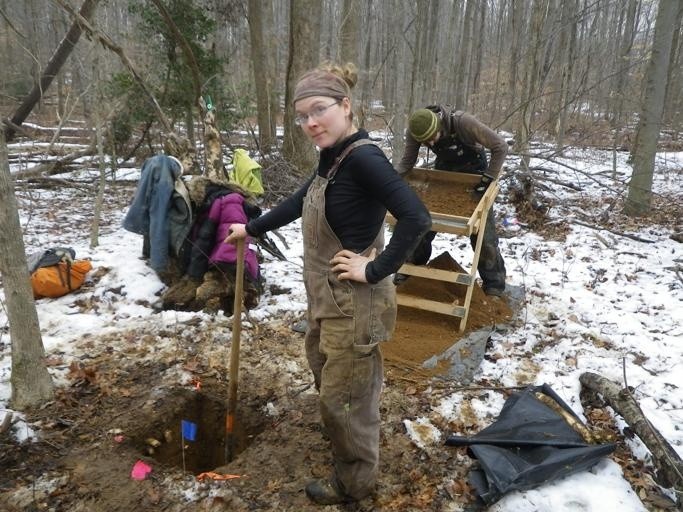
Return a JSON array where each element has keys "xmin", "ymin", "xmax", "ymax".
[{"xmin": 306, "ymin": 476, "xmax": 370, "ymax": 503}]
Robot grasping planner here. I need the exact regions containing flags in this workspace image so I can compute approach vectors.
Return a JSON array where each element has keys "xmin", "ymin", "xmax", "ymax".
[{"xmin": 182, "ymin": 420, "xmax": 197, "ymax": 441}]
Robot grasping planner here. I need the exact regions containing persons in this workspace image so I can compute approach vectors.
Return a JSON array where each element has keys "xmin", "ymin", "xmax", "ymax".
[
  {"xmin": 223, "ymin": 59, "xmax": 431, "ymax": 507},
  {"xmin": 395, "ymin": 106, "xmax": 509, "ymax": 296}
]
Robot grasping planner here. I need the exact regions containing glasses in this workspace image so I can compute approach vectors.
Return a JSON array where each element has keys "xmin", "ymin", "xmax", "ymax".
[{"xmin": 293, "ymin": 102, "xmax": 340, "ymax": 125}]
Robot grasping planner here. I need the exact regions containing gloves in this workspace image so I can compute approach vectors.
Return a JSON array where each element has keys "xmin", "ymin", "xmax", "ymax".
[{"xmin": 475, "ymin": 173, "xmax": 495, "ymax": 196}]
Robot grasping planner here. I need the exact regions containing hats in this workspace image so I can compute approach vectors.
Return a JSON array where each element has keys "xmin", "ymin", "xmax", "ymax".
[{"xmin": 408, "ymin": 110, "xmax": 439, "ymax": 143}]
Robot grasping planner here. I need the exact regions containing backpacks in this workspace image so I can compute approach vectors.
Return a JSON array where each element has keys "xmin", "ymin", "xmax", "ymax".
[{"xmin": 23, "ymin": 247, "xmax": 90, "ymax": 299}]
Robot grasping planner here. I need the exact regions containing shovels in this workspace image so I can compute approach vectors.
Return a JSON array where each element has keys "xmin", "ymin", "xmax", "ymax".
[{"xmin": 204, "ymin": 240, "xmax": 244, "ymax": 466}]
